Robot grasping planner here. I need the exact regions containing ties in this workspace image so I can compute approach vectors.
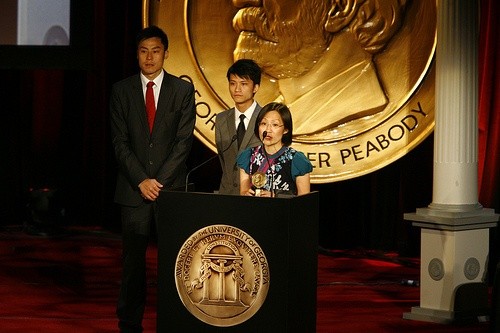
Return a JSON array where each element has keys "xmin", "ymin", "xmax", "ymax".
[
  {"xmin": 236, "ymin": 114, "xmax": 247, "ymax": 149},
  {"xmin": 145, "ymin": 82, "xmax": 157, "ymax": 134}
]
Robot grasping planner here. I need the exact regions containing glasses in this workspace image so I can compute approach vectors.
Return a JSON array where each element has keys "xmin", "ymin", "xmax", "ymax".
[{"xmin": 258, "ymin": 121, "xmax": 285, "ymax": 129}]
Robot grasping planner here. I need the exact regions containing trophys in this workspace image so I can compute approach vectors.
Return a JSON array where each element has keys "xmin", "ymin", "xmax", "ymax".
[{"xmin": 252, "ymin": 171, "xmax": 267, "ymax": 197}]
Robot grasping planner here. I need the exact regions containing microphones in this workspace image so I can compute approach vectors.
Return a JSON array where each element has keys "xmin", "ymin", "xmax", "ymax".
[
  {"xmin": 185, "ymin": 135, "xmax": 238, "ymax": 191},
  {"xmin": 262, "ymin": 131, "xmax": 273, "ymax": 198}
]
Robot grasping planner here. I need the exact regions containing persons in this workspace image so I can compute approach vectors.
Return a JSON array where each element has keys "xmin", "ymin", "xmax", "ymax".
[
  {"xmin": 237, "ymin": 104, "xmax": 312, "ymax": 197},
  {"xmin": 108, "ymin": 25, "xmax": 196, "ymax": 332},
  {"xmin": 214, "ymin": 59, "xmax": 264, "ymax": 196}
]
